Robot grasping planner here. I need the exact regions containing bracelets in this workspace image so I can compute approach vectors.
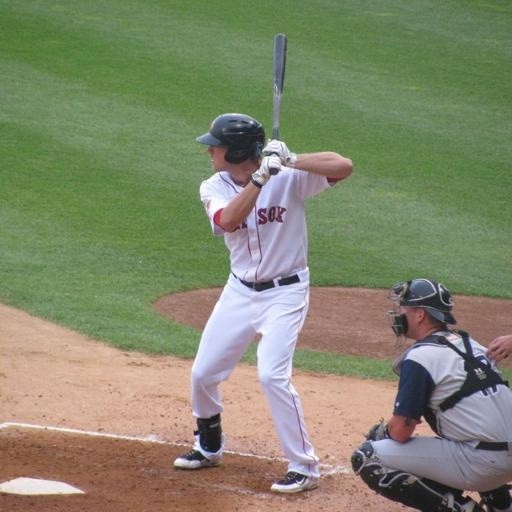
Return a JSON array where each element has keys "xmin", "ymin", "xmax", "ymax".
[{"xmin": 251, "ymin": 178, "xmax": 263, "ymax": 189}]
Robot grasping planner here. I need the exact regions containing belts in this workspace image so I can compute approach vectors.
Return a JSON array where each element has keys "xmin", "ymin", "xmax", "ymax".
[
  {"xmin": 475, "ymin": 440, "xmax": 509, "ymax": 451},
  {"xmin": 230, "ymin": 272, "xmax": 300, "ymax": 293}
]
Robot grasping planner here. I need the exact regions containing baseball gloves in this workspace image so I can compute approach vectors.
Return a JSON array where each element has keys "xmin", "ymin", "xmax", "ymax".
[{"xmin": 366, "ymin": 423, "xmax": 390, "ymax": 440}]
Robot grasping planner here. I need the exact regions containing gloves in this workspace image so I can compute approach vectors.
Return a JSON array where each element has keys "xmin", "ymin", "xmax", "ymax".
[{"xmin": 251, "ymin": 139, "xmax": 297, "ymax": 187}]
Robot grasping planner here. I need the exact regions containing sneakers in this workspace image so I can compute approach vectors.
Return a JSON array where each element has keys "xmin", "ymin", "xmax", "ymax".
[
  {"xmin": 270, "ymin": 471, "xmax": 320, "ymax": 495},
  {"xmin": 173, "ymin": 432, "xmax": 227, "ymax": 470}
]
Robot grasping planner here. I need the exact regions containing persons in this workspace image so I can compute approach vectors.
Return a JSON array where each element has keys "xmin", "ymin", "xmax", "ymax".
[
  {"xmin": 173, "ymin": 114, "xmax": 353, "ymax": 494},
  {"xmin": 351, "ymin": 279, "xmax": 512, "ymax": 512},
  {"xmin": 485, "ymin": 334, "xmax": 512, "ymax": 369}
]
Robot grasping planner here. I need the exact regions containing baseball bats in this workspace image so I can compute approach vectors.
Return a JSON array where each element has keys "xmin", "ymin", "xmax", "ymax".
[{"xmin": 270, "ymin": 33, "xmax": 287, "ymax": 175}]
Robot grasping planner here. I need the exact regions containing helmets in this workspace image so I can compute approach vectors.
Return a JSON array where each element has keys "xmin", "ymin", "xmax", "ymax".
[
  {"xmin": 194, "ymin": 112, "xmax": 266, "ymax": 166},
  {"xmin": 388, "ymin": 278, "xmax": 457, "ymax": 350}
]
